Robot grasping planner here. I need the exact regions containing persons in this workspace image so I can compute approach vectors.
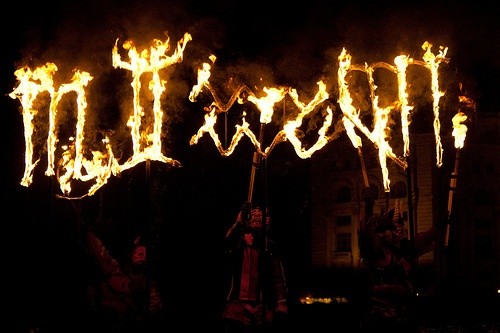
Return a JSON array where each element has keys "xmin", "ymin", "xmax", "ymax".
[
  {"xmin": 358, "ymin": 184, "xmax": 438, "ymax": 333},
  {"xmin": 79, "ymin": 223, "xmax": 164, "ymax": 333},
  {"xmin": 219, "ymin": 203, "xmax": 288, "ymax": 333}
]
